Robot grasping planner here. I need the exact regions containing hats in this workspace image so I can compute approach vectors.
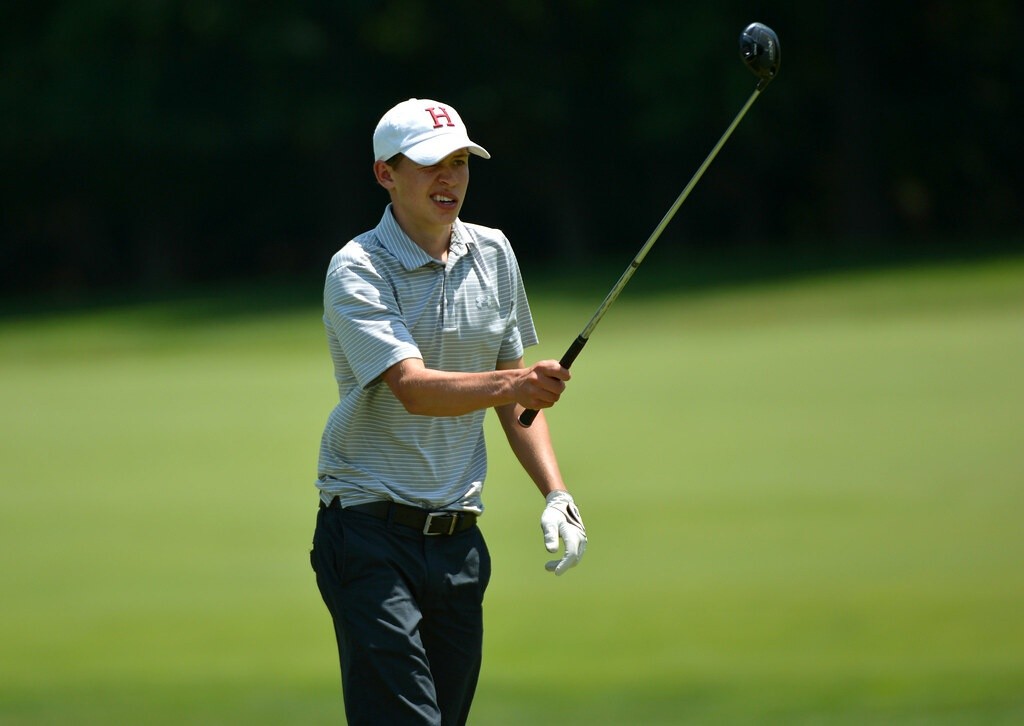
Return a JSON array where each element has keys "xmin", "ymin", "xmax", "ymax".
[{"xmin": 372, "ymin": 96, "xmax": 492, "ymax": 165}]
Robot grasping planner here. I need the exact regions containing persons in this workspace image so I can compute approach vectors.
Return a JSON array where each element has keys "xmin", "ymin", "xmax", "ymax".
[{"xmin": 305, "ymin": 94, "xmax": 591, "ymax": 726}]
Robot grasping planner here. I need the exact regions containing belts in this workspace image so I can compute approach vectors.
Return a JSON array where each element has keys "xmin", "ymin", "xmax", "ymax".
[{"xmin": 318, "ymin": 495, "xmax": 480, "ymax": 536}]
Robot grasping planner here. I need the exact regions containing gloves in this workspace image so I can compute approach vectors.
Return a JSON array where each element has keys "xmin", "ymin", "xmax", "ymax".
[{"xmin": 538, "ymin": 489, "xmax": 587, "ymax": 576}]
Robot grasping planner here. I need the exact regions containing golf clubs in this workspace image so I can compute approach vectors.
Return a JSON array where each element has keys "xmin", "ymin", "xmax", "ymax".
[{"xmin": 518, "ymin": 21, "xmax": 780, "ymax": 428}]
